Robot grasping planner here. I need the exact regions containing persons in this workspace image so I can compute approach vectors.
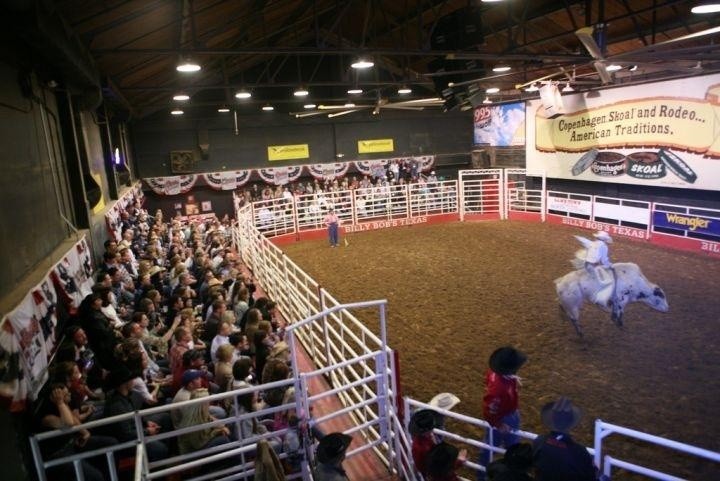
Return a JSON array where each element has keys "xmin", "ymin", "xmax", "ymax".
[
  {"xmin": 408, "ymin": 347, "xmax": 600, "ymax": 481},
  {"xmin": 569, "ymin": 231, "xmax": 616, "ymax": 284}
]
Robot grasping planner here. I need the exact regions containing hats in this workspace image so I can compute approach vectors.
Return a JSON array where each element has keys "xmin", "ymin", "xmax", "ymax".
[
  {"xmin": 408, "ymin": 409, "xmax": 442, "ymax": 433},
  {"xmin": 430, "ymin": 392, "xmax": 460, "ymax": 410},
  {"xmin": 181, "ymin": 369, "xmax": 208, "ymax": 384},
  {"xmin": 504, "ymin": 442, "xmax": 533, "ymax": 468},
  {"xmin": 540, "ymin": 396, "xmax": 582, "ymax": 432},
  {"xmin": 102, "ymin": 366, "xmax": 137, "ymax": 392},
  {"xmin": 317, "ymin": 432, "xmax": 353, "ymax": 464},
  {"xmin": 148, "ymin": 265, "xmax": 166, "ymax": 277},
  {"xmin": 489, "ymin": 347, "xmax": 527, "ymax": 375},
  {"xmin": 593, "ymin": 230, "xmax": 613, "ymax": 244}
]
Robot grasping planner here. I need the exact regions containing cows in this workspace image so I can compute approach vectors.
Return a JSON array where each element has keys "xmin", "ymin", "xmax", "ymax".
[{"xmin": 551, "ymin": 262, "xmax": 670, "ymax": 339}]
